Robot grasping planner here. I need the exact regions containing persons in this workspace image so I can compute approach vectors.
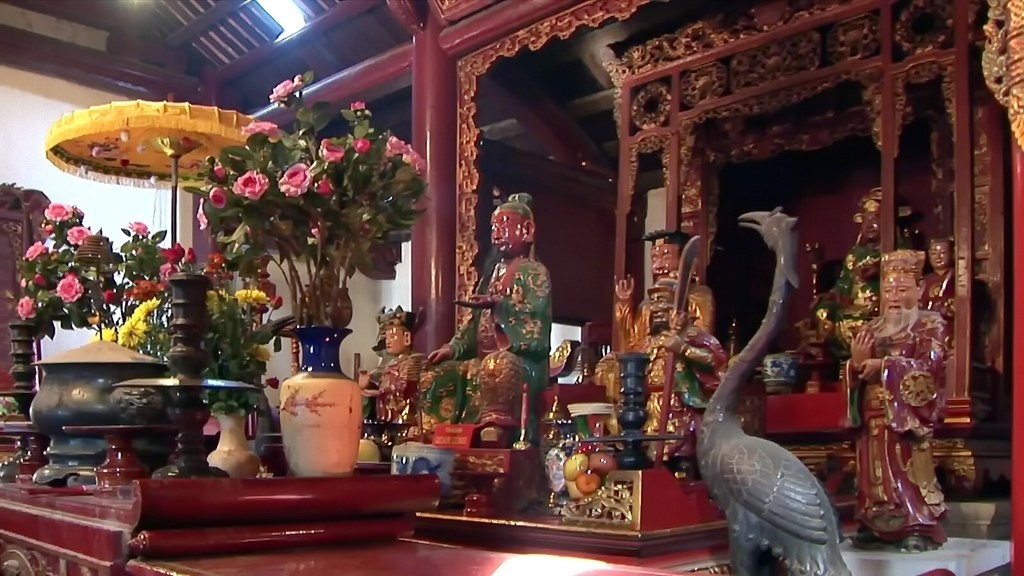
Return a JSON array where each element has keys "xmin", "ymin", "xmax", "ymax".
[
  {"xmin": 845, "ymin": 250, "xmax": 947, "ymax": 553},
  {"xmin": 421, "ymin": 193, "xmax": 552, "ymax": 448},
  {"xmin": 813, "ymin": 186, "xmax": 956, "ymax": 358},
  {"xmin": 593, "ymin": 231, "xmax": 728, "ymax": 471},
  {"xmin": 359, "ymin": 305, "xmax": 426, "ymax": 446}
]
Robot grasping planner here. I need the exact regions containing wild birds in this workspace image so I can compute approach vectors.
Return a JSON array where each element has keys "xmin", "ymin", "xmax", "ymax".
[{"xmin": 696, "ymin": 206, "xmax": 853, "ymax": 576}]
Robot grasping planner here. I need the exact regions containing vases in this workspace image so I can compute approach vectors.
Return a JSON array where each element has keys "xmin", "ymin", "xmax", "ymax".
[
  {"xmin": 211, "ymin": 412, "xmax": 261, "ymax": 479},
  {"xmin": 279, "ymin": 326, "xmax": 362, "ymax": 477}
]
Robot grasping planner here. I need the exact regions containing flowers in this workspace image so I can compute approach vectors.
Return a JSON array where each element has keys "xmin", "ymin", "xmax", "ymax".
[{"xmin": 16, "ymin": 70, "xmax": 432, "ymax": 419}]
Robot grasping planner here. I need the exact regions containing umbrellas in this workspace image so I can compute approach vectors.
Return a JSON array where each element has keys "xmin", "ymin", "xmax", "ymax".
[{"xmin": 45, "ymin": 99, "xmax": 261, "ymax": 248}]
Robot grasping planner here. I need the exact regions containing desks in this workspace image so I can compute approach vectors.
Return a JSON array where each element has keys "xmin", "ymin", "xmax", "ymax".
[{"xmin": 0, "ymin": 474, "xmax": 858, "ymax": 576}]
